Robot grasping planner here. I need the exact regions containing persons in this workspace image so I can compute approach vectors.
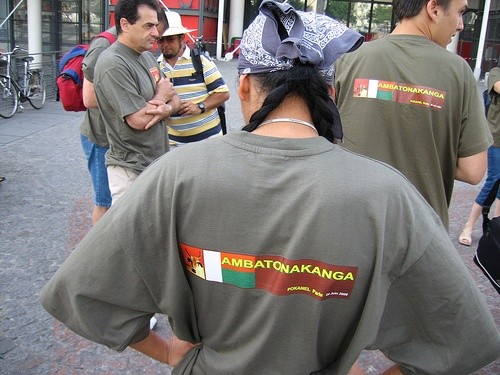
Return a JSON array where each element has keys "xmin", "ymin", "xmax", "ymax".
[
  {"xmin": 458, "ymin": 67, "xmax": 500, "ymax": 245},
  {"xmin": 331, "ymin": 0, "xmax": 494, "ymax": 233},
  {"xmin": 94, "ymin": 0, "xmax": 181, "ymax": 330},
  {"xmin": 38, "ymin": 0, "xmax": 500, "ymax": 375},
  {"xmin": 155, "ymin": 11, "xmax": 230, "ymax": 148},
  {"xmin": 80, "ymin": 7, "xmax": 169, "ymax": 223}
]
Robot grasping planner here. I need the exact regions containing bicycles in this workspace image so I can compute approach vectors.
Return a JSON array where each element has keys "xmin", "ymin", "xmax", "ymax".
[
  {"xmin": 0, "ymin": 46, "xmax": 46, "ymax": 119},
  {"xmin": 191, "ymin": 35, "xmax": 208, "ymax": 54}
]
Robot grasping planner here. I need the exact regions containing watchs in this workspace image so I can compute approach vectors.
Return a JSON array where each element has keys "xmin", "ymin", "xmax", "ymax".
[{"xmin": 197, "ymin": 102, "xmax": 205, "ymax": 114}]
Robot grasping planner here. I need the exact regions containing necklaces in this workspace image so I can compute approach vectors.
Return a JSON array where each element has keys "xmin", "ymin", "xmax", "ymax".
[{"xmin": 259, "ymin": 118, "xmax": 318, "ymax": 133}]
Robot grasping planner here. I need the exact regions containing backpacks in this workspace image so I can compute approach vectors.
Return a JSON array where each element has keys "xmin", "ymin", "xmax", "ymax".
[{"xmin": 55, "ymin": 32, "xmax": 115, "ymax": 111}]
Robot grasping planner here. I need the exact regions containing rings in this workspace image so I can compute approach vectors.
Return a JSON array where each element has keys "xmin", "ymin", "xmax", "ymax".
[{"xmin": 186, "ymin": 107, "xmax": 188, "ymax": 111}]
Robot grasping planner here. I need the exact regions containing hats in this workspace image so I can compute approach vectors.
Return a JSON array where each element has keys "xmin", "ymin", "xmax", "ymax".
[{"xmin": 160, "ymin": 11, "xmax": 198, "ymax": 36}]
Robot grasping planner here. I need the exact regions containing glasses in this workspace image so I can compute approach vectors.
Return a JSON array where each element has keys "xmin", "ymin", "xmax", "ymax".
[{"xmin": 157, "ymin": 36, "xmax": 179, "ymax": 44}]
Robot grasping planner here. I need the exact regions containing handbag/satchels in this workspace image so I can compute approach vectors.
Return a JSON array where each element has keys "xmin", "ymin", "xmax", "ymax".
[{"xmin": 474, "ymin": 220, "xmax": 500, "ymax": 295}]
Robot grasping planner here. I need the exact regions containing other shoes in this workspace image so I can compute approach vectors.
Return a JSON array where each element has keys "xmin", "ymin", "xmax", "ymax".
[{"xmin": 459, "ymin": 230, "xmax": 472, "ymax": 246}]
